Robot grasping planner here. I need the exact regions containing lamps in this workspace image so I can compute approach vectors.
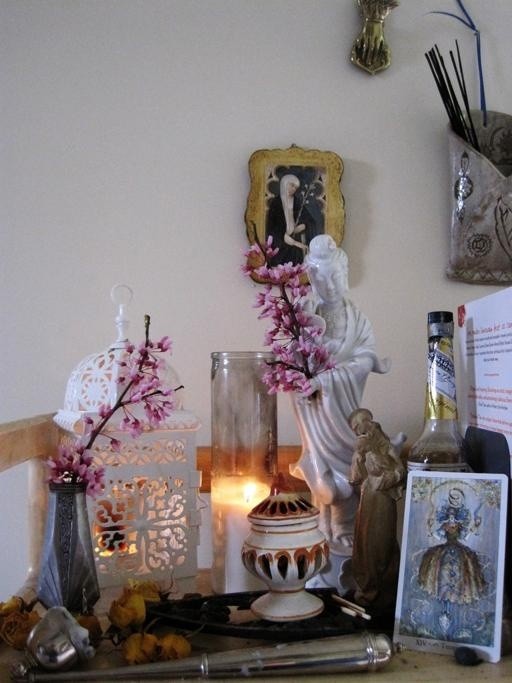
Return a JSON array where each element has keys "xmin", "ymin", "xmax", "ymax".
[{"xmin": 53, "ymin": 284, "xmax": 208, "ymax": 618}]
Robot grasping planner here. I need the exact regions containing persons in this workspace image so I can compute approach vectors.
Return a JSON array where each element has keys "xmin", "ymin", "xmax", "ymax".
[
  {"xmin": 348, "ymin": 407, "xmax": 408, "ymax": 591},
  {"xmin": 418, "ymin": 509, "xmax": 490, "ymax": 606},
  {"xmin": 266, "ymin": 174, "xmax": 315, "ymax": 268},
  {"xmin": 282, "ymin": 233, "xmax": 394, "ymax": 549}
]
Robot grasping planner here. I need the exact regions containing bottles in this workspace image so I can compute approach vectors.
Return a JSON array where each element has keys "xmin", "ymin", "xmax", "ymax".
[
  {"xmin": 212, "ymin": 350, "xmax": 277, "ymax": 597},
  {"xmin": 405, "ymin": 311, "xmax": 472, "ymax": 473}
]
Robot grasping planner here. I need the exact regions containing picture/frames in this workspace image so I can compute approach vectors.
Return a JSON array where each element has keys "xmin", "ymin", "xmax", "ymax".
[{"xmin": 242, "ymin": 142, "xmax": 346, "ymax": 291}]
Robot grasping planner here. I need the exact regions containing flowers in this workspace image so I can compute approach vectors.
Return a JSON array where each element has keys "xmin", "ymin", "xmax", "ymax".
[
  {"xmin": 42, "ymin": 315, "xmax": 187, "ymax": 501},
  {"xmin": 238, "ymin": 221, "xmax": 340, "ymax": 407}
]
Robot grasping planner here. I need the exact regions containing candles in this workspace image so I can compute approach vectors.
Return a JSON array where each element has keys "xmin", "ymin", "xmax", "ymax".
[{"xmin": 211, "ymin": 475, "xmax": 271, "ymax": 595}]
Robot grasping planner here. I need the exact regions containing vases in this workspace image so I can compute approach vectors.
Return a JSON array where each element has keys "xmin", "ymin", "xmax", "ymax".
[{"xmin": 36, "ymin": 477, "xmax": 101, "ymax": 614}]
